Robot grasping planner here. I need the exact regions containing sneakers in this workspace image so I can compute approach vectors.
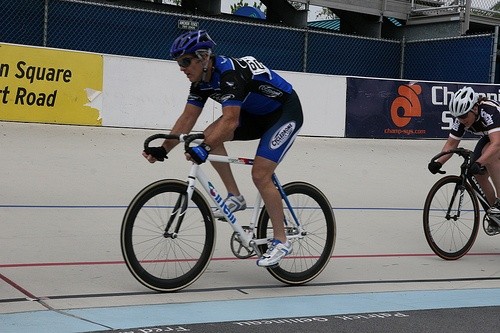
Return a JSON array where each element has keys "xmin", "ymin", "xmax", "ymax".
[
  {"xmin": 212, "ymin": 193, "xmax": 247, "ymax": 217},
  {"xmin": 256, "ymin": 239, "xmax": 293, "ymax": 267},
  {"xmin": 487, "ymin": 198, "xmax": 500, "ymax": 213}
]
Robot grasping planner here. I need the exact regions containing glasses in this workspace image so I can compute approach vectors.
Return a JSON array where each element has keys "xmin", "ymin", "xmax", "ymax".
[
  {"xmin": 176, "ymin": 51, "xmax": 209, "ymax": 67},
  {"xmin": 457, "ymin": 103, "xmax": 476, "ymax": 118}
]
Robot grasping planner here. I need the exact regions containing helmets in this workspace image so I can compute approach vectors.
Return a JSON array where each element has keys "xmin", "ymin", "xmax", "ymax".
[
  {"xmin": 449, "ymin": 86, "xmax": 477, "ymax": 117},
  {"xmin": 170, "ymin": 29, "xmax": 217, "ymax": 59}
]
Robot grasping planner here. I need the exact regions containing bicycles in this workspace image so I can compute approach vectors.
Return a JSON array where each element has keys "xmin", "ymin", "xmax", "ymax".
[
  {"xmin": 421, "ymin": 145, "xmax": 500, "ymax": 262},
  {"xmin": 119, "ymin": 132, "xmax": 338, "ymax": 292}
]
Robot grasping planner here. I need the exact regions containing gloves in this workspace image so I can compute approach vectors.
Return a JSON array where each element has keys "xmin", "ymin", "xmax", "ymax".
[
  {"xmin": 428, "ymin": 161, "xmax": 441, "ymax": 175},
  {"xmin": 466, "ymin": 162, "xmax": 481, "ymax": 177}
]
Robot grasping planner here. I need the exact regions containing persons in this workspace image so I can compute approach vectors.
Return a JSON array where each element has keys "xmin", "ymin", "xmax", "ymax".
[
  {"xmin": 141, "ymin": 28, "xmax": 304, "ymax": 267},
  {"xmin": 428, "ymin": 86, "xmax": 500, "ymax": 231}
]
